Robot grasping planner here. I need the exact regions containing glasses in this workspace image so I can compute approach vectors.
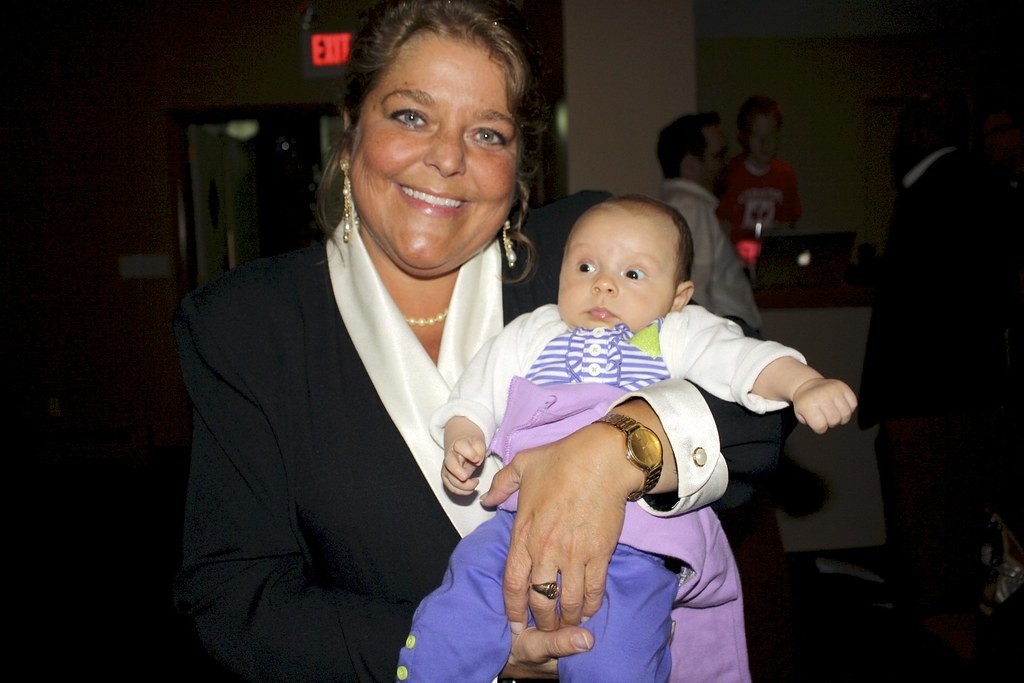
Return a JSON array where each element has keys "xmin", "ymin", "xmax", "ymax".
[{"xmin": 980, "ymin": 122, "xmax": 1019, "ymax": 139}]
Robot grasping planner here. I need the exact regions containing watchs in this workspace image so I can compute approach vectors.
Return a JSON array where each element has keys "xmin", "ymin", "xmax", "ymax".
[{"xmin": 591, "ymin": 413, "xmax": 663, "ymax": 502}]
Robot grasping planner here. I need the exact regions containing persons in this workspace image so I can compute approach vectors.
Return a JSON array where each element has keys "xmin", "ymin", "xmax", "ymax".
[
  {"xmin": 858, "ymin": 91, "xmax": 1024, "ymax": 683},
  {"xmin": 170, "ymin": 0, "xmax": 859, "ymax": 683}
]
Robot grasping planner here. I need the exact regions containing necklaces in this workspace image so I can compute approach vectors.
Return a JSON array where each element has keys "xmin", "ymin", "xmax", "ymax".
[{"xmin": 405, "ymin": 311, "xmax": 449, "ymax": 327}]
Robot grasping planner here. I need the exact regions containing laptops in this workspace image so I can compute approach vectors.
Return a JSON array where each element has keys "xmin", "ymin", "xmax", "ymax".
[{"xmin": 753, "ymin": 230, "xmax": 859, "ymax": 291}]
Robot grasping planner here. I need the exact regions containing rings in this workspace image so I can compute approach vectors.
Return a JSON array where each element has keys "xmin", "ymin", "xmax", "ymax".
[{"xmin": 532, "ymin": 582, "xmax": 559, "ymax": 599}]
